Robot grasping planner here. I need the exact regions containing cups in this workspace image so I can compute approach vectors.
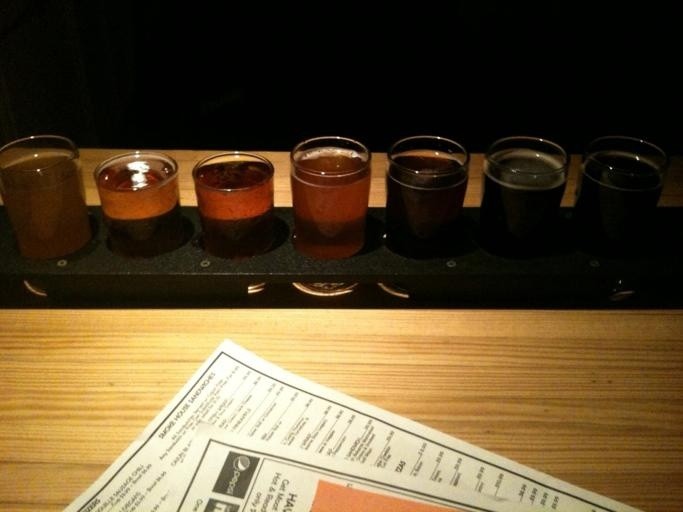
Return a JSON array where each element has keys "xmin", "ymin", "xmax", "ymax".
[
  {"xmin": 3, "ymin": 134, "xmax": 93, "ymax": 260},
  {"xmin": 382, "ymin": 134, "xmax": 473, "ymax": 260},
  {"xmin": 478, "ymin": 136, "xmax": 573, "ymax": 257},
  {"xmin": 568, "ymin": 134, "xmax": 665, "ymax": 253},
  {"xmin": 290, "ymin": 136, "xmax": 372, "ymax": 257},
  {"xmin": 97, "ymin": 151, "xmax": 186, "ymax": 261},
  {"xmin": 193, "ymin": 153, "xmax": 278, "ymax": 258}
]
